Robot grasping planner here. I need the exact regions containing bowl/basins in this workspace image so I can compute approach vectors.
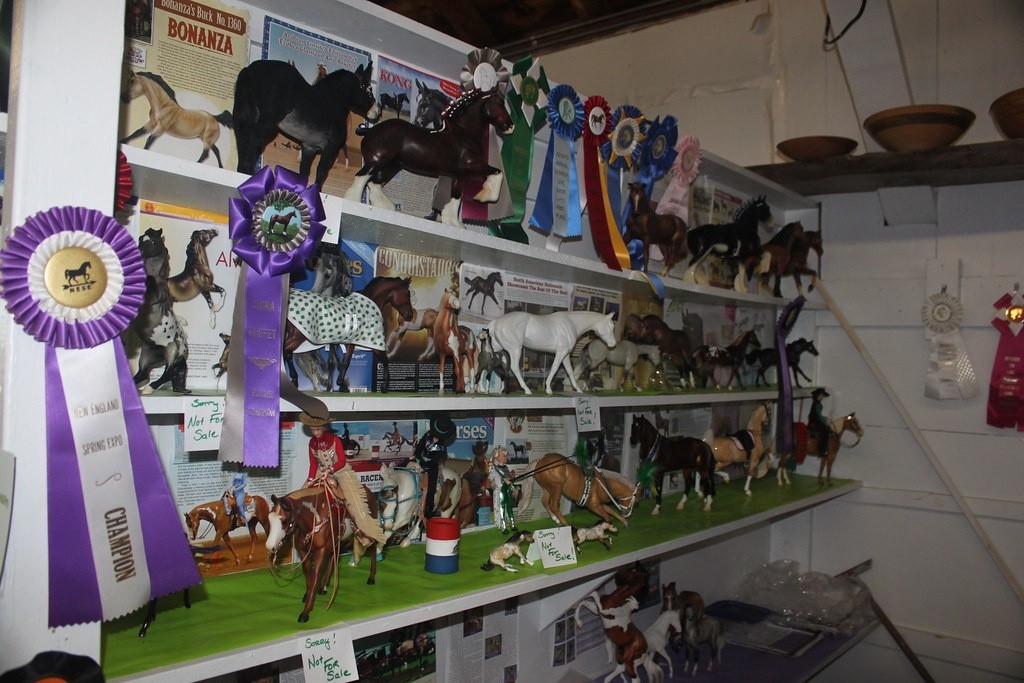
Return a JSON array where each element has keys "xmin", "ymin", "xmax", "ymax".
[
  {"xmin": 989, "ymin": 86, "xmax": 1024, "ymax": 139},
  {"xmin": 863, "ymin": 103, "xmax": 977, "ymax": 154}
]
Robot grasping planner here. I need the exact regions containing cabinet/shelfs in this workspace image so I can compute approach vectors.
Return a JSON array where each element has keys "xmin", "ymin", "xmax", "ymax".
[{"xmin": 100, "ymin": 17, "xmax": 883, "ymax": 683}]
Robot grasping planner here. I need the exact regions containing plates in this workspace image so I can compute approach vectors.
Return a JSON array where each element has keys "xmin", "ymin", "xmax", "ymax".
[
  {"xmin": 775, "ymin": 135, "xmax": 859, "ymax": 163},
  {"xmin": 704, "ymin": 600, "xmax": 771, "ymax": 624}
]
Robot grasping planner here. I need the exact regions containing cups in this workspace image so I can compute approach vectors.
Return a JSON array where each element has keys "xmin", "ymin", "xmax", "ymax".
[{"xmin": 424, "ymin": 518, "xmax": 460, "ymax": 573}]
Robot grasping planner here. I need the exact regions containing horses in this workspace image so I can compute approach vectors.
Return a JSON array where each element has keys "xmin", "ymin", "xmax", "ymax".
[
  {"xmin": 183, "ymin": 495, "xmax": 269, "ymax": 565},
  {"xmin": 340, "ymin": 87, "xmax": 515, "ymax": 224},
  {"xmin": 462, "ymin": 271, "xmax": 503, "ymax": 315},
  {"xmin": 432, "ymin": 288, "xmax": 476, "ymax": 395},
  {"xmin": 282, "ymin": 276, "xmax": 413, "ymax": 393},
  {"xmin": 168, "ymin": 230, "xmax": 232, "ymax": 378},
  {"xmin": 263, "ymin": 413, "xmax": 864, "ymax": 623},
  {"xmin": 119, "ymin": 69, "xmax": 232, "ymax": 169},
  {"xmin": 385, "ymin": 305, "xmax": 438, "ymax": 360},
  {"xmin": 234, "ymin": 59, "xmax": 381, "ymax": 194},
  {"xmin": 295, "ymin": 241, "xmax": 352, "ymax": 392},
  {"xmin": 65, "ymin": 262, "xmax": 92, "ymax": 286},
  {"xmin": 562, "ymin": 314, "xmax": 819, "ymax": 394},
  {"xmin": 378, "ymin": 78, "xmax": 452, "ymax": 130},
  {"xmin": 475, "ymin": 328, "xmax": 510, "ymax": 395},
  {"xmin": 127, "ymin": 228, "xmax": 191, "ymax": 395},
  {"xmin": 622, "ymin": 182, "xmax": 823, "ymax": 303},
  {"xmin": 485, "ymin": 311, "xmax": 618, "ymax": 395},
  {"xmin": 574, "ymin": 559, "xmax": 726, "ymax": 683},
  {"xmin": 266, "ymin": 210, "xmax": 298, "ymax": 235}
]
